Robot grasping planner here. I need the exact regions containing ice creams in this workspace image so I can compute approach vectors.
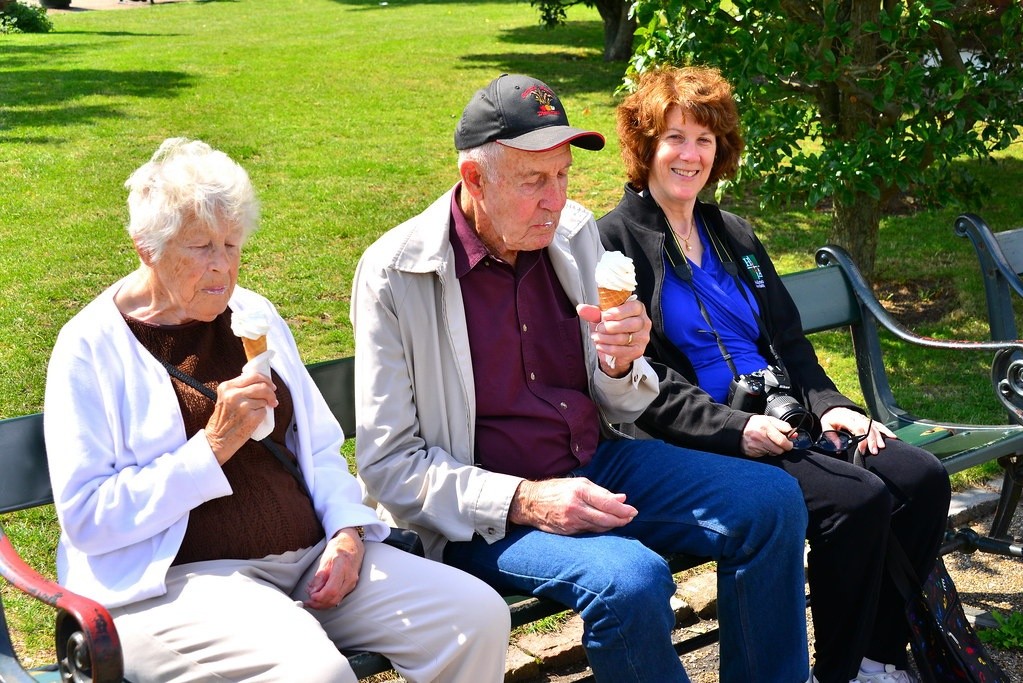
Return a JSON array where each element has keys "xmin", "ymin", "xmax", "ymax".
[
  {"xmin": 595, "ymin": 250, "xmax": 637, "ymax": 312},
  {"xmin": 230, "ymin": 296, "xmax": 270, "ymax": 361}
]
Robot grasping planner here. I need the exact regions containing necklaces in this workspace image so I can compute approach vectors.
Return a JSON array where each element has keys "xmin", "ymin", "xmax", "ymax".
[{"xmin": 674, "ymin": 216, "xmax": 694, "ymax": 251}]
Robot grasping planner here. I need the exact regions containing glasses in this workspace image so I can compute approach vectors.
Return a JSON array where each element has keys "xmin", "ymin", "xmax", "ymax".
[{"xmin": 785, "ymin": 407, "xmax": 873, "ymax": 453}]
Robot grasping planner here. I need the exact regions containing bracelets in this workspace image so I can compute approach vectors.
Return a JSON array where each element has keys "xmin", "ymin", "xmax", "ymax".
[{"xmin": 356, "ymin": 526, "xmax": 365, "ymax": 541}]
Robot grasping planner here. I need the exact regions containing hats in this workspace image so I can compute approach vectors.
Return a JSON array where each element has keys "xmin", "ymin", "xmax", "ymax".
[{"xmin": 454, "ymin": 73, "xmax": 606, "ymax": 153}]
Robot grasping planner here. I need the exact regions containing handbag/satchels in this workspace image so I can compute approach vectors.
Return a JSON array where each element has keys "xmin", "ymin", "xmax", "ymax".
[{"xmin": 904, "ymin": 556, "xmax": 1009, "ymax": 683}]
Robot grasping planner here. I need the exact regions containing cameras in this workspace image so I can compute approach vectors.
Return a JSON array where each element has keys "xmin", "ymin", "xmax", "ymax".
[{"xmin": 729, "ymin": 365, "xmax": 813, "ymax": 436}]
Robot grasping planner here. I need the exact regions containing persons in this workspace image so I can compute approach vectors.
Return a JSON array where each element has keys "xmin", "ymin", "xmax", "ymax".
[
  {"xmin": 349, "ymin": 75, "xmax": 811, "ymax": 683},
  {"xmin": 595, "ymin": 65, "xmax": 952, "ymax": 683},
  {"xmin": 44, "ymin": 137, "xmax": 511, "ymax": 683}
]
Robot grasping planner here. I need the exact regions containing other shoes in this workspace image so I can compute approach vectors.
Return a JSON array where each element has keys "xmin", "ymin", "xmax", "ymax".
[
  {"xmin": 806, "ymin": 666, "xmax": 861, "ymax": 683},
  {"xmin": 857, "ymin": 664, "xmax": 917, "ymax": 683}
]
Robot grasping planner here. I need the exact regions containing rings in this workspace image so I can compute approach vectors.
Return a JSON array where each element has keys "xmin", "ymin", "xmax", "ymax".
[
  {"xmin": 336, "ymin": 603, "xmax": 339, "ymax": 608},
  {"xmin": 866, "ymin": 418, "xmax": 870, "ymax": 421},
  {"xmin": 766, "ymin": 450, "xmax": 769, "ymax": 455},
  {"xmin": 626, "ymin": 332, "xmax": 633, "ymax": 344}
]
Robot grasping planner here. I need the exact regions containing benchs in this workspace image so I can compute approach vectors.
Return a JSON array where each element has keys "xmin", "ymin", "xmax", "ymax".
[
  {"xmin": 953, "ymin": 212, "xmax": 1023, "ymax": 543},
  {"xmin": 0, "ymin": 243, "xmax": 1023, "ymax": 683}
]
